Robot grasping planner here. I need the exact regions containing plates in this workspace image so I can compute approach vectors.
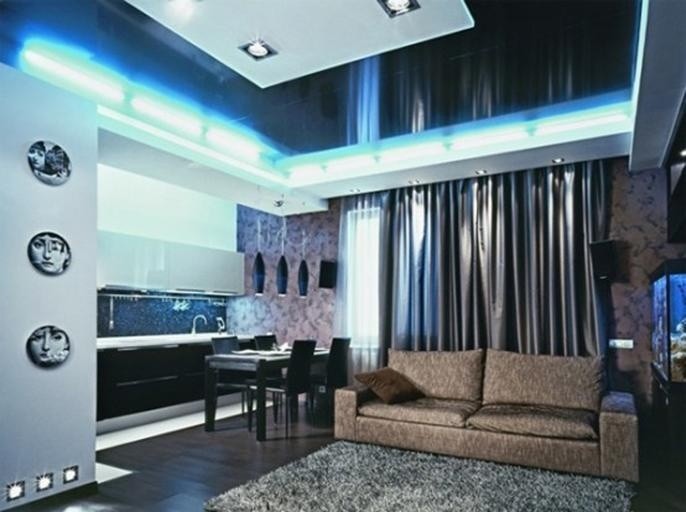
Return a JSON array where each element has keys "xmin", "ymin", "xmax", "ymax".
[
  {"xmin": 314, "ymin": 346, "xmax": 327, "ymax": 351},
  {"xmin": 231, "ymin": 349, "xmax": 292, "ymax": 356}
]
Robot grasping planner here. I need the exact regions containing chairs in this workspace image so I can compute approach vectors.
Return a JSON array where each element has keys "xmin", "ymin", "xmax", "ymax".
[{"xmin": 211, "ymin": 332, "xmax": 352, "ymax": 437}]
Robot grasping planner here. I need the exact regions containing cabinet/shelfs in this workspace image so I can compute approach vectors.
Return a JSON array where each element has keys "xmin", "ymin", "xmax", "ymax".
[{"xmin": 96, "ymin": 345, "xmax": 207, "ymax": 421}]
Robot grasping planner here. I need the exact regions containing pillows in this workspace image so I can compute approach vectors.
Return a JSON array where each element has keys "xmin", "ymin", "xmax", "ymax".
[{"xmin": 349, "ymin": 365, "xmax": 429, "ymax": 406}]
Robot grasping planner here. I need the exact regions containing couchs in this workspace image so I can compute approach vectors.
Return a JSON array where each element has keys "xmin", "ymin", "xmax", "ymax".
[{"xmin": 335, "ymin": 346, "xmax": 644, "ymax": 485}]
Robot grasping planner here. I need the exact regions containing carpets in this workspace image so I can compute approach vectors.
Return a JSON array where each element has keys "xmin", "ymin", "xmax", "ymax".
[{"xmin": 202, "ymin": 440, "xmax": 639, "ymax": 512}]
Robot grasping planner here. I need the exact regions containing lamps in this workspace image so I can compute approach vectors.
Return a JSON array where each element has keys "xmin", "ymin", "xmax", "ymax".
[{"xmin": 251, "ymin": 184, "xmax": 312, "ymax": 299}]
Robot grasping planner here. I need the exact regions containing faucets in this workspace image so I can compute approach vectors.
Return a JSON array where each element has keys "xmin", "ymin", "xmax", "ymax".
[{"xmin": 191, "ymin": 314, "xmax": 207, "ymax": 334}]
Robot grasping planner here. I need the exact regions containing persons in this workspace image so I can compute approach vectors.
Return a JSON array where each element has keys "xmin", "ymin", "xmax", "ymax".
[
  {"xmin": 27, "ymin": 231, "xmax": 71, "ymax": 276},
  {"xmin": 25, "ymin": 325, "xmax": 69, "ymax": 370},
  {"xmin": 26, "ymin": 138, "xmax": 68, "ymax": 186}
]
